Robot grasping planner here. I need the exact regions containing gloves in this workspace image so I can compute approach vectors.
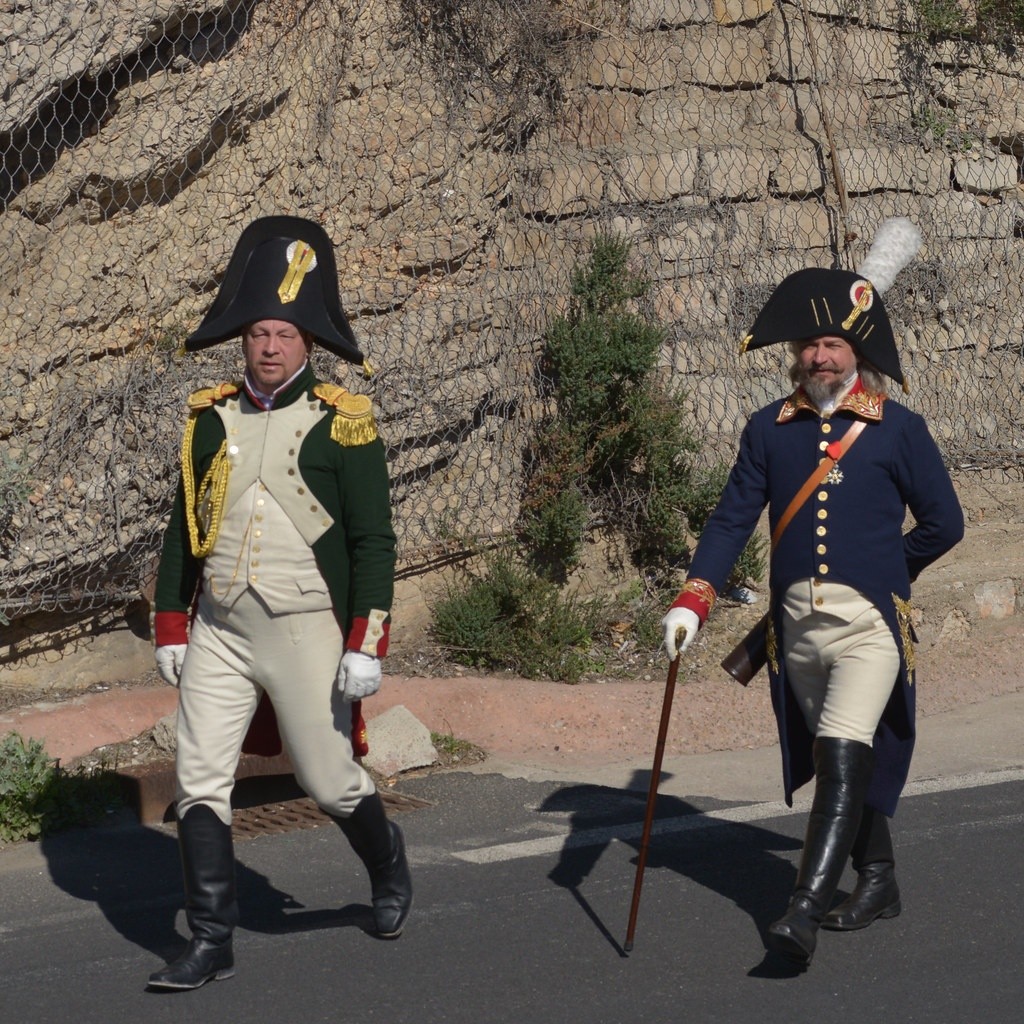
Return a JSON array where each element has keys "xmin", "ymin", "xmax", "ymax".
[
  {"xmin": 155, "ymin": 644, "xmax": 188, "ymax": 688},
  {"xmin": 338, "ymin": 649, "xmax": 381, "ymax": 705},
  {"xmin": 661, "ymin": 607, "xmax": 700, "ymax": 661}
]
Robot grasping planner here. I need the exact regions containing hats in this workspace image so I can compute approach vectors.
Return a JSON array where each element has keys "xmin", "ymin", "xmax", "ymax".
[
  {"xmin": 186, "ymin": 214, "xmax": 364, "ymax": 365},
  {"xmin": 740, "ymin": 267, "xmax": 910, "ymax": 396}
]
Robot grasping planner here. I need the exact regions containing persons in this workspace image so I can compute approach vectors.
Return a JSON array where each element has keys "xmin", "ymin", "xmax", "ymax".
[
  {"xmin": 144, "ymin": 213, "xmax": 414, "ymax": 992},
  {"xmin": 661, "ymin": 267, "xmax": 964, "ymax": 974}
]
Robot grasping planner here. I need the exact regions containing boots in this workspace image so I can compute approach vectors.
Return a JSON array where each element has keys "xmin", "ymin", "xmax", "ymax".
[
  {"xmin": 148, "ymin": 804, "xmax": 237, "ymax": 989},
  {"xmin": 319, "ymin": 788, "xmax": 415, "ymax": 940},
  {"xmin": 822, "ymin": 803, "xmax": 902, "ymax": 931},
  {"xmin": 769, "ymin": 736, "xmax": 874, "ymax": 974}
]
